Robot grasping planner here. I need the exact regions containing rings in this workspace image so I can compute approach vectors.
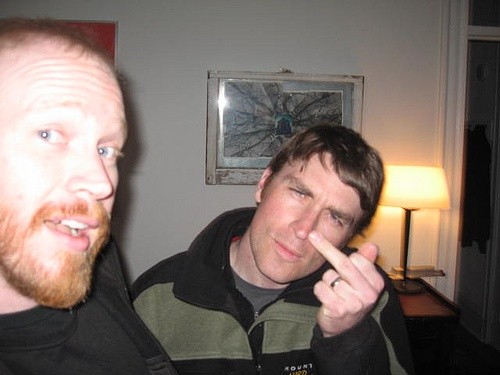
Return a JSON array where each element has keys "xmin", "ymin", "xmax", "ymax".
[{"xmin": 328, "ymin": 275, "xmax": 342, "ymax": 287}]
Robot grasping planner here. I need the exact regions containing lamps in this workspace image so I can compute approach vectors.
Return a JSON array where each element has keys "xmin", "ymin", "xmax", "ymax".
[{"xmin": 378, "ymin": 165, "xmax": 450, "ymax": 279}]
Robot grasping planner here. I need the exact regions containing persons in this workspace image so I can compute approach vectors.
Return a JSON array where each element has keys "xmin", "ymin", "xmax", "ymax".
[
  {"xmin": 127, "ymin": 123, "xmax": 417, "ymax": 375},
  {"xmin": 1, "ymin": 17, "xmax": 178, "ymax": 375}
]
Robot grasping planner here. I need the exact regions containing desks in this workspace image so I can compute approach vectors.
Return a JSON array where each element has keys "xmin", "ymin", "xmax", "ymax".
[{"xmin": 391, "ymin": 277, "xmax": 461, "ymax": 375}]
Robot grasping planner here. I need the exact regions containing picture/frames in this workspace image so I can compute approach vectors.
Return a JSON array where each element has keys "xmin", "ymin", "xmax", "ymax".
[{"xmin": 205, "ymin": 69, "xmax": 364, "ymax": 185}]
